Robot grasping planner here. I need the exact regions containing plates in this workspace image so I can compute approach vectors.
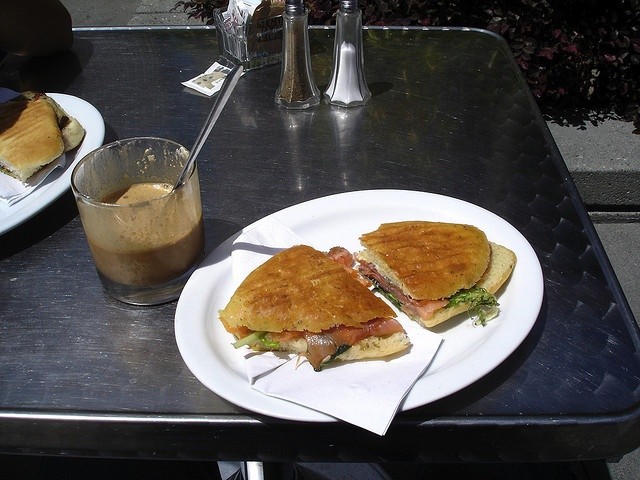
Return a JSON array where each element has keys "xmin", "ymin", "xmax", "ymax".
[
  {"xmin": 2, "ymin": 91, "xmax": 106, "ymax": 239},
  {"xmin": 175, "ymin": 189, "xmax": 543, "ymax": 421}
]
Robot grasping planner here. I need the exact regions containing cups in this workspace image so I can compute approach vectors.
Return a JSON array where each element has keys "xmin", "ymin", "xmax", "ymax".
[
  {"xmin": 213, "ymin": 6, "xmax": 285, "ymax": 71},
  {"xmin": 70, "ymin": 134, "xmax": 210, "ymax": 306}
]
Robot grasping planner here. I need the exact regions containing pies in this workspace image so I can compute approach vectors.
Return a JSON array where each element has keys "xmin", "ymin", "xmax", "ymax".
[
  {"xmin": 0, "ymin": 89, "xmax": 87, "ymax": 188},
  {"xmin": 216, "ymin": 243, "xmax": 413, "ymax": 371},
  {"xmin": 353, "ymin": 218, "xmax": 517, "ymax": 332}
]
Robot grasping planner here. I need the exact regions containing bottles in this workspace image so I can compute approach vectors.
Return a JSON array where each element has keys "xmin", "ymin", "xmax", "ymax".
[
  {"xmin": 271, "ymin": 0, "xmax": 322, "ymax": 113},
  {"xmin": 323, "ymin": 1, "xmax": 373, "ymax": 108}
]
